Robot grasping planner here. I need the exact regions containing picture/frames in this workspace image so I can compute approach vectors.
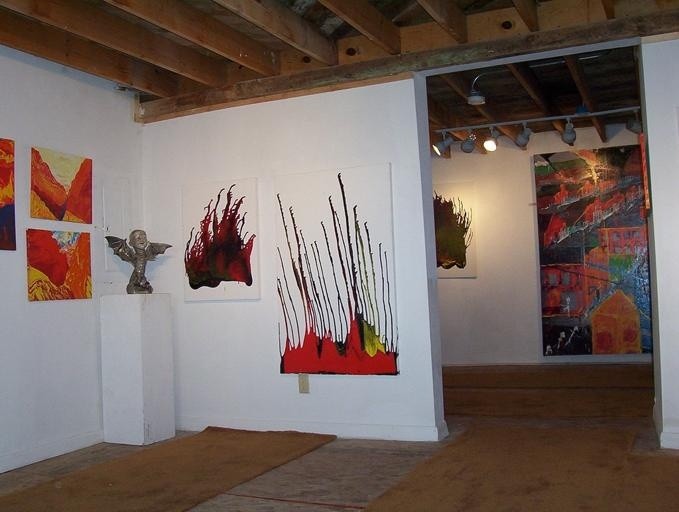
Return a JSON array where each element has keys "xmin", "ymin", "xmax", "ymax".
[{"xmin": 530, "ymin": 142, "xmax": 653, "ymax": 364}]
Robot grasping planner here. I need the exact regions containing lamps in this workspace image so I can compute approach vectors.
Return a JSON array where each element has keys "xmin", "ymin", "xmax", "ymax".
[
  {"xmin": 467, "ymin": 70, "xmax": 488, "ymax": 105},
  {"xmin": 430, "ymin": 106, "xmax": 644, "ymax": 156}
]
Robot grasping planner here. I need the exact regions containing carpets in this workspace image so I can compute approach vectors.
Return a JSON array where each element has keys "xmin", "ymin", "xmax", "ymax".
[
  {"xmin": 360, "ymin": 415, "xmax": 679, "ymax": 512},
  {"xmin": 0, "ymin": 425, "xmax": 336, "ymax": 512}
]
